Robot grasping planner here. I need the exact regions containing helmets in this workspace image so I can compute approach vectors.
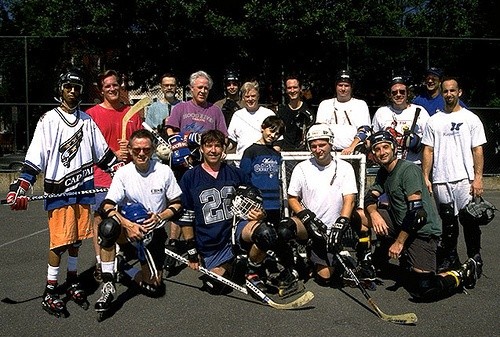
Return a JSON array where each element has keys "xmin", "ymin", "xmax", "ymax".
[
  {"xmin": 462, "ymin": 195, "xmax": 496, "ymax": 226},
  {"xmin": 427, "ymin": 67, "xmax": 444, "ymax": 81},
  {"xmin": 392, "ymin": 77, "xmax": 407, "ymax": 86},
  {"xmin": 306, "ymin": 124, "xmax": 334, "ymax": 151},
  {"xmin": 58, "ymin": 72, "xmax": 84, "ymax": 95},
  {"xmin": 227, "ymin": 185, "xmax": 263, "ymax": 220},
  {"xmin": 370, "ymin": 131, "xmax": 395, "ymax": 163},
  {"xmin": 336, "ymin": 70, "xmax": 353, "ymax": 86},
  {"xmin": 224, "ymin": 71, "xmax": 240, "ymax": 84}
]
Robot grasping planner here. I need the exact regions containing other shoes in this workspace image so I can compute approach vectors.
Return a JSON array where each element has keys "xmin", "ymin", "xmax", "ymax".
[
  {"xmin": 245, "ymin": 273, "xmax": 279, "ymax": 295},
  {"xmin": 93, "ymin": 263, "xmax": 102, "ymax": 281}
]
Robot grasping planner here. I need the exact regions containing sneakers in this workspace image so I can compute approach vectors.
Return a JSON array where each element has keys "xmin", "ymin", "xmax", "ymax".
[
  {"xmin": 468, "ymin": 254, "xmax": 483, "ymax": 278},
  {"xmin": 438, "ymin": 257, "xmax": 460, "ymax": 272}
]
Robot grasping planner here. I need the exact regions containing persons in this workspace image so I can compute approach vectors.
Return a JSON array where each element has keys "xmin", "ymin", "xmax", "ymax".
[
  {"xmin": 5, "ymin": 69, "xmax": 487, "ymax": 312},
  {"xmin": 175, "ymin": 130, "xmax": 276, "ymax": 294},
  {"xmin": 94, "ymin": 129, "xmax": 184, "ymax": 312},
  {"xmin": 3, "ymin": 69, "xmax": 125, "ymax": 311},
  {"xmin": 352, "ymin": 129, "xmax": 477, "ymax": 301},
  {"xmin": 276, "ymin": 125, "xmax": 362, "ymax": 288},
  {"xmin": 240, "ymin": 115, "xmax": 285, "ymax": 224}
]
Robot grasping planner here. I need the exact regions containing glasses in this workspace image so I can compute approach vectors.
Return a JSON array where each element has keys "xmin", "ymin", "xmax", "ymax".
[{"xmin": 130, "ymin": 146, "xmax": 152, "ymax": 154}]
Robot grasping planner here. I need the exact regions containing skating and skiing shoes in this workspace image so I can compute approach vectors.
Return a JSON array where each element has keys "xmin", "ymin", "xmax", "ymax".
[
  {"xmin": 164, "ymin": 239, "xmax": 180, "ymax": 278},
  {"xmin": 65, "ymin": 278, "xmax": 88, "ymax": 309},
  {"xmin": 278, "ymin": 268, "xmax": 306, "ymax": 297},
  {"xmin": 462, "ymin": 257, "xmax": 477, "ymax": 289},
  {"xmin": 94, "ymin": 272, "xmax": 117, "ymax": 321},
  {"xmin": 343, "ymin": 264, "xmax": 378, "ymax": 291},
  {"xmin": 41, "ymin": 281, "xmax": 67, "ymax": 318},
  {"xmin": 114, "ymin": 254, "xmax": 125, "ymax": 283}
]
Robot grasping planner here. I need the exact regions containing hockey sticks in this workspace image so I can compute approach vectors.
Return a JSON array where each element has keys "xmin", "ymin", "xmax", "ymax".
[
  {"xmin": 140, "ymin": 121, "xmax": 193, "ymax": 169},
  {"xmin": 300, "ymin": 199, "xmax": 419, "ymax": 322},
  {"xmin": 0, "ymin": 186, "xmax": 109, "ymax": 206},
  {"xmin": 246, "ymin": 278, "xmax": 315, "ymax": 312},
  {"xmin": 121, "ymin": 97, "xmax": 151, "ymax": 139},
  {"xmin": 166, "ymin": 248, "xmax": 247, "ymax": 293}
]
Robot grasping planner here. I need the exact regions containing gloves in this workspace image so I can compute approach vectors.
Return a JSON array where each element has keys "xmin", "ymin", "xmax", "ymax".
[
  {"xmin": 7, "ymin": 178, "xmax": 31, "ymax": 210},
  {"xmin": 168, "ymin": 134, "xmax": 191, "ymax": 166},
  {"xmin": 402, "ymin": 130, "xmax": 422, "ymax": 153},
  {"xmin": 297, "ymin": 208, "xmax": 327, "ymax": 238},
  {"xmin": 328, "ymin": 217, "xmax": 350, "ymax": 253}
]
